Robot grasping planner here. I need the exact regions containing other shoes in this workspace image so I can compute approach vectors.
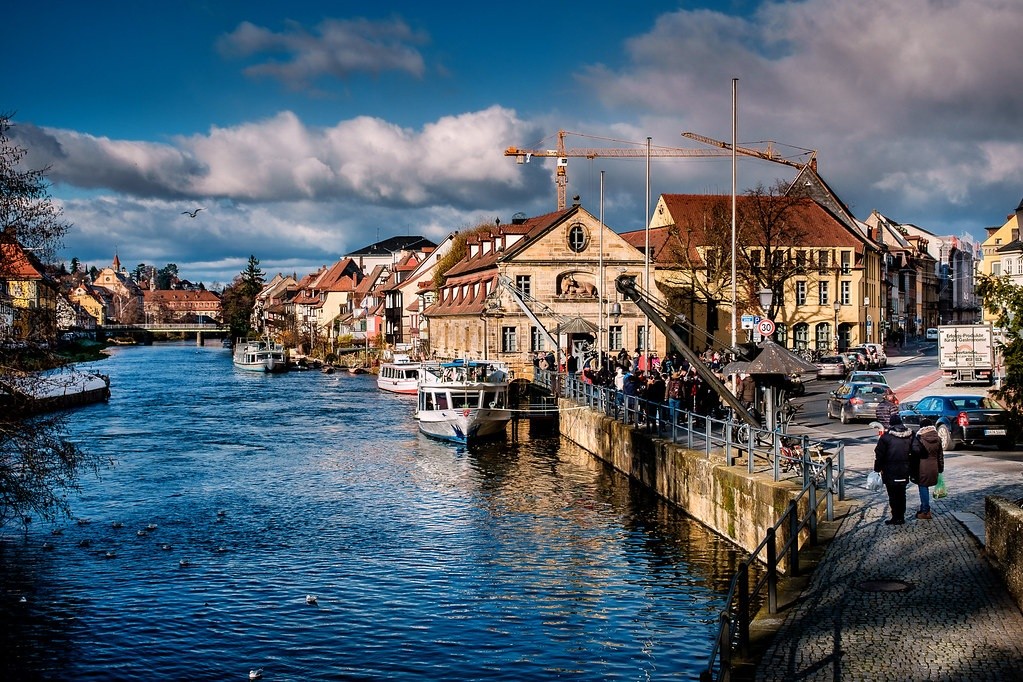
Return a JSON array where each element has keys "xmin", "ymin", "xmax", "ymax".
[{"xmin": 885, "ymin": 517, "xmax": 905, "ymax": 525}]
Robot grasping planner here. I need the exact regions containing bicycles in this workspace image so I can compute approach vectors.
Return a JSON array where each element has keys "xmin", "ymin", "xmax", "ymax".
[{"xmin": 722, "ymin": 402, "xmax": 839, "ymax": 495}]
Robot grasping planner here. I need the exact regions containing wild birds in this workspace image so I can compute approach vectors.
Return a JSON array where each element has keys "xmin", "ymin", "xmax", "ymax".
[
  {"xmin": 306, "ymin": 594, "xmax": 318, "ymax": 604},
  {"xmin": 26, "ymin": 509, "xmax": 231, "ymax": 567},
  {"xmin": 180, "ymin": 208, "xmax": 207, "ymax": 219},
  {"xmin": 248, "ymin": 669, "xmax": 263, "ymax": 680}
]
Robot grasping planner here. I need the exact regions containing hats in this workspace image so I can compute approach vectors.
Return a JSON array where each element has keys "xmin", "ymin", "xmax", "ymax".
[
  {"xmin": 671, "ymin": 373, "xmax": 682, "ymax": 378},
  {"xmin": 889, "ymin": 415, "xmax": 902, "ymax": 426}
]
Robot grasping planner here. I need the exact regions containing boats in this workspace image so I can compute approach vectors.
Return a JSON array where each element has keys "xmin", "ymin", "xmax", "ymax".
[
  {"xmin": 376, "ymin": 359, "xmax": 446, "ymax": 395},
  {"xmin": 0, "ymin": 366, "xmax": 111, "ymax": 417},
  {"xmin": 231, "ymin": 335, "xmax": 287, "ymax": 373},
  {"xmin": 414, "ymin": 353, "xmax": 513, "ymax": 445}
]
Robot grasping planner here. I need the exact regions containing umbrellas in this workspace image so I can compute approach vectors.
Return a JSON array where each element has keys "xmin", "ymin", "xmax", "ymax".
[{"xmin": 716, "ymin": 334, "xmax": 819, "ymax": 375}]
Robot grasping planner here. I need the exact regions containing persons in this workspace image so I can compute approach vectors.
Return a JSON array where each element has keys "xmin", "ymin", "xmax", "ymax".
[
  {"xmin": 875, "ymin": 415, "xmax": 915, "ymax": 524},
  {"xmin": 910, "ymin": 419, "xmax": 944, "ymax": 519},
  {"xmin": 531, "ymin": 346, "xmax": 756, "ymax": 434}
]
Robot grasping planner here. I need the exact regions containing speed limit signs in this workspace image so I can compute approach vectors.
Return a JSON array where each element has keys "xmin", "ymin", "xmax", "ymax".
[{"xmin": 757, "ymin": 319, "xmax": 775, "ymax": 336}]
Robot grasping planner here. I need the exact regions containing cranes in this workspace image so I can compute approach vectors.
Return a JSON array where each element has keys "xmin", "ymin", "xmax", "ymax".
[
  {"xmin": 680, "ymin": 131, "xmax": 819, "ymax": 170},
  {"xmin": 502, "ymin": 129, "xmax": 783, "ymax": 213}
]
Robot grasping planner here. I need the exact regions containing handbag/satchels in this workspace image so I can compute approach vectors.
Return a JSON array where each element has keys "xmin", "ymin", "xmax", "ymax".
[
  {"xmin": 933, "ymin": 473, "xmax": 947, "ymax": 499},
  {"xmin": 866, "ymin": 471, "xmax": 883, "ymax": 492}
]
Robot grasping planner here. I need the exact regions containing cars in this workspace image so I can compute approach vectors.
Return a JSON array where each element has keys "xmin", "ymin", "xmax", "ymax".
[
  {"xmin": 896, "ymin": 394, "xmax": 1017, "ymax": 451},
  {"xmin": 826, "ymin": 380, "xmax": 899, "ymax": 425},
  {"xmin": 787, "ymin": 321, "xmax": 1007, "ymax": 387},
  {"xmin": 837, "ymin": 370, "xmax": 888, "ymax": 385}
]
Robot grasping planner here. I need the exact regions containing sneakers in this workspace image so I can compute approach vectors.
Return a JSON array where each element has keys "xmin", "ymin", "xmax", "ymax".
[{"xmin": 916, "ymin": 511, "xmax": 930, "ymax": 519}]
